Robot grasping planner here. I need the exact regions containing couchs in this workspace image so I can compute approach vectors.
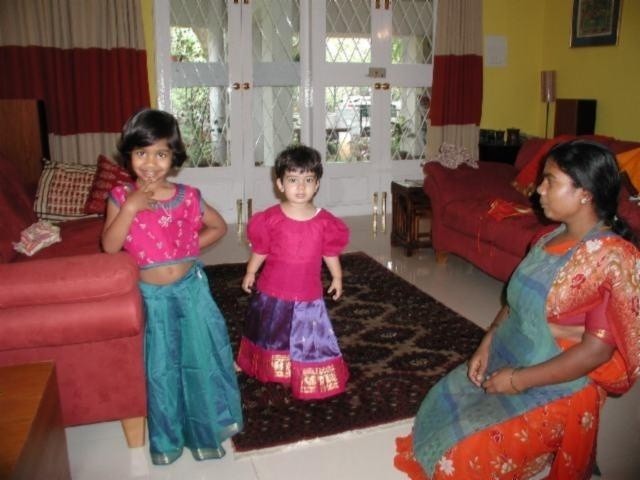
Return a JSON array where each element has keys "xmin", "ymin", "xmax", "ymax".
[
  {"xmin": 422, "ymin": 136, "xmax": 640, "ymax": 284},
  {"xmin": 0, "ymin": 154, "xmax": 148, "ymax": 448}
]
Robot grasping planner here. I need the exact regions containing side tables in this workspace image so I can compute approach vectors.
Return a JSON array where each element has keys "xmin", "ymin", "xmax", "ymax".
[{"xmin": 390, "ymin": 178, "xmax": 432, "ymax": 257}]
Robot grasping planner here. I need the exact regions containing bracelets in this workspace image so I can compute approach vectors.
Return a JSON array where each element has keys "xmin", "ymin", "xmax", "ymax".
[{"xmin": 509, "ymin": 367, "xmax": 528, "ymax": 396}]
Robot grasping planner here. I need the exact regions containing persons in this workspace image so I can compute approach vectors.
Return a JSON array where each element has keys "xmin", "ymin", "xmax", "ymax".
[
  {"xmin": 393, "ymin": 138, "xmax": 640, "ymax": 480},
  {"xmin": 103, "ymin": 109, "xmax": 245, "ymax": 467},
  {"xmin": 241, "ymin": 145, "xmax": 350, "ymax": 402}
]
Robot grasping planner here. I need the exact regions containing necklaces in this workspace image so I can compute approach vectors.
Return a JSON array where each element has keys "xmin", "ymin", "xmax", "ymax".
[{"xmin": 144, "ymin": 180, "xmax": 176, "ymax": 223}]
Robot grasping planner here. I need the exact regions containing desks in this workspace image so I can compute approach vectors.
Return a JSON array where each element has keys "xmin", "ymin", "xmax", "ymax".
[{"xmin": 1, "ymin": 361, "xmax": 72, "ymax": 480}]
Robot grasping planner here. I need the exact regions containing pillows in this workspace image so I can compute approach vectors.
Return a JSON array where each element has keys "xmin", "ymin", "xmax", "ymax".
[{"xmin": 84, "ymin": 154, "xmax": 136, "ymax": 215}]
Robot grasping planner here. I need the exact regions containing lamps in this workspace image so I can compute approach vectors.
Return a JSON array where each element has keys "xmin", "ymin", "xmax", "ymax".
[{"xmin": 540, "ymin": 71, "xmax": 556, "ymax": 139}]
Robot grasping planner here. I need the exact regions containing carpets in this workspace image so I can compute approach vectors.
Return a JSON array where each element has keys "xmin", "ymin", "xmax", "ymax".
[{"xmin": 201, "ymin": 250, "xmax": 488, "ymax": 463}]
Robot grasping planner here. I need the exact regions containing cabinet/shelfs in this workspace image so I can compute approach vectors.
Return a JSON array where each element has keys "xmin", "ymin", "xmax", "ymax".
[{"xmin": 0, "ymin": 98, "xmax": 51, "ymax": 189}]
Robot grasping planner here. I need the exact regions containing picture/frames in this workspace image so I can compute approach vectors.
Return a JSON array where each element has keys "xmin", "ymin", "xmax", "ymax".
[{"xmin": 569, "ymin": 0, "xmax": 623, "ymax": 48}]
